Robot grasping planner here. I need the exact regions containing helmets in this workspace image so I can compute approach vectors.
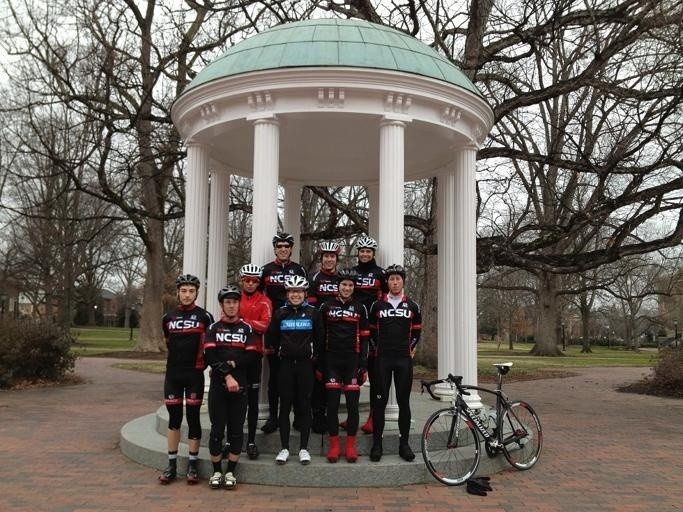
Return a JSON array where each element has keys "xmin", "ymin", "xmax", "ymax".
[
  {"xmin": 284, "ymin": 275, "xmax": 309, "ymax": 292},
  {"xmin": 336, "ymin": 268, "xmax": 358, "ymax": 284},
  {"xmin": 319, "ymin": 241, "xmax": 341, "ymax": 255},
  {"xmin": 385, "ymin": 264, "xmax": 405, "ymax": 280},
  {"xmin": 239, "ymin": 264, "xmax": 263, "ymax": 279},
  {"xmin": 272, "ymin": 233, "xmax": 294, "ymax": 247},
  {"xmin": 218, "ymin": 285, "xmax": 241, "ymax": 305},
  {"xmin": 175, "ymin": 274, "xmax": 200, "ymax": 290},
  {"xmin": 355, "ymin": 236, "xmax": 377, "ymax": 251}
]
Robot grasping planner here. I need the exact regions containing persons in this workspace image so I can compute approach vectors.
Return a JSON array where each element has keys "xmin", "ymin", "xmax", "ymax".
[
  {"xmin": 204, "ymin": 284, "xmax": 257, "ymax": 489},
  {"xmin": 220, "ymin": 263, "xmax": 273, "ymax": 459},
  {"xmin": 258, "ymin": 232, "xmax": 308, "ymax": 434},
  {"xmin": 338, "ymin": 235, "xmax": 386, "ymax": 432},
  {"xmin": 368, "ymin": 264, "xmax": 421, "ymax": 462},
  {"xmin": 308, "ymin": 242, "xmax": 356, "ymax": 432},
  {"xmin": 264, "ymin": 274, "xmax": 319, "ymax": 464},
  {"xmin": 157, "ymin": 273, "xmax": 215, "ymax": 484},
  {"xmin": 319, "ymin": 265, "xmax": 369, "ymax": 461}
]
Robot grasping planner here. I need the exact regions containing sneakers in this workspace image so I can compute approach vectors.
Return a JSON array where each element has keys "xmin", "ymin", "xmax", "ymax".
[
  {"xmin": 159, "ymin": 464, "xmax": 176, "ymax": 483},
  {"xmin": 186, "ymin": 463, "xmax": 199, "ymax": 485},
  {"xmin": 276, "ymin": 449, "xmax": 289, "ymax": 465},
  {"xmin": 246, "ymin": 441, "xmax": 258, "ymax": 460},
  {"xmin": 299, "ymin": 449, "xmax": 311, "ymax": 465},
  {"xmin": 360, "ymin": 418, "xmax": 373, "ymax": 434},
  {"xmin": 221, "ymin": 443, "xmax": 230, "ymax": 458},
  {"xmin": 370, "ymin": 442, "xmax": 383, "ymax": 462},
  {"xmin": 399, "ymin": 441, "xmax": 415, "ymax": 462},
  {"xmin": 209, "ymin": 471, "xmax": 236, "ymax": 490},
  {"xmin": 261, "ymin": 416, "xmax": 279, "ymax": 433},
  {"xmin": 293, "ymin": 417, "xmax": 348, "ymax": 433}
]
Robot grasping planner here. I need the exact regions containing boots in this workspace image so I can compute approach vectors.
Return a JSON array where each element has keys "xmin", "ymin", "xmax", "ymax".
[
  {"xmin": 327, "ymin": 435, "xmax": 341, "ymax": 463},
  {"xmin": 344, "ymin": 436, "xmax": 357, "ymax": 463}
]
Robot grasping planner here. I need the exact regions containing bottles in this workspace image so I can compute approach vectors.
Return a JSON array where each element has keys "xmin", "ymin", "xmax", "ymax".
[{"xmin": 474, "ymin": 408, "xmax": 489, "ymax": 425}]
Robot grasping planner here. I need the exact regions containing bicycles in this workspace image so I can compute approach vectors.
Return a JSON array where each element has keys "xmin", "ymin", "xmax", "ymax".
[{"xmin": 418, "ymin": 360, "xmax": 544, "ymax": 486}]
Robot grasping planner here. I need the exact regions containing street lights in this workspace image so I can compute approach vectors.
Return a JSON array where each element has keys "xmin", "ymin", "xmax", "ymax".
[
  {"xmin": 612, "ymin": 330, "xmax": 615, "ymax": 344},
  {"xmin": 129, "ymin": 304, "xmax": 138, "ymax": 341},
  {"xmin": 674, "ymin": 319, "xmax": 679, "ymax": 348},
  {"xmin": 561, "ymin": 323, "xmax": 567, "ymax": 351}
]
